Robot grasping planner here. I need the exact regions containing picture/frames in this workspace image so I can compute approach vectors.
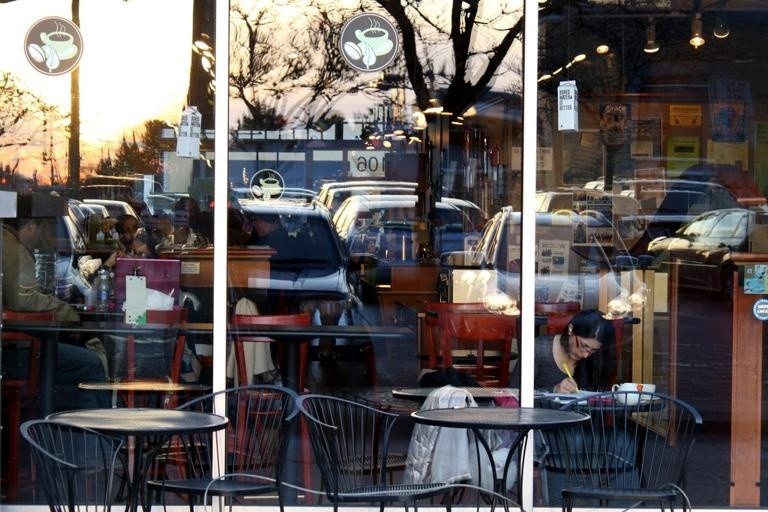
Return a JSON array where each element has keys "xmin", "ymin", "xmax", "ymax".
[{"xmin": 630, "ymin": 115, "xmax": 663, "ymax": 161}]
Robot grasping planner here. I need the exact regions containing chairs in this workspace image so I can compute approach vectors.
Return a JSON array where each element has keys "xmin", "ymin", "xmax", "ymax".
[
  {"xmin": 297, "ymin": 387, "xmax": 452, "ymax": 512},
  {"xmin": 144, "ymin": 384, "xmax": 296, "ymax": 511},
  {"xmin": 19, "ymin": 418, "xmax": 127, "ymax": 512},
  {"xmin": 558, "ymin": 392, "xmax": 702, "ymax": 512},
  {"xmin": 6, "ymin": 299, "xmax": 674, "ymax": 506}
]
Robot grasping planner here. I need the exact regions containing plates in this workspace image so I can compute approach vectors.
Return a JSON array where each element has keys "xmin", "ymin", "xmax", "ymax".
[{"xmin": 641, "ymin": 397, "xmax": 661, "ymax": 402}]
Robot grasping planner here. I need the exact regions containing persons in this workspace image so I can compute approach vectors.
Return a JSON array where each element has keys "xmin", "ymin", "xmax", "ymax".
[
  {"xmin": 508, "ymin": 309, "xmax": 616, "ymax": 506},
  {"xmin": 0, "ymin": 212, "xmax": 123, "ymax": 465},
  {"xmin": 104, "ymin": 198, "xmax": 294, "ymax": 271}
]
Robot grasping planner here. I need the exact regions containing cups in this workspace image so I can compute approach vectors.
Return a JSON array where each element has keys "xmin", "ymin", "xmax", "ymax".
[{"xmin": 641, "ymin": 383, "xmax": 656, "ymax": 397}]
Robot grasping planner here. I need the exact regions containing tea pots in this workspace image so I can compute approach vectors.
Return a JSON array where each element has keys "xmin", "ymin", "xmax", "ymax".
[{"xmin": 612, "ymin": 382, "xmax": 646, "ymax": 405}]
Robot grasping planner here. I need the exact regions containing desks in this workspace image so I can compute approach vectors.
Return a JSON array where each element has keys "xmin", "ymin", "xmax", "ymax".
[
  {"xmin": 339, "ymin": 243, "xmax": 452, "ymax": 305},
  {"xmin": 157, "ymin": 243, "xmax": 279, "ymax": 290},
  {"xmin": 407, "ymin": 405, "xmax": 592, "ymax": 512},
  {"xmin": 43, "ymin": 405, "xmax": 228, "ymax": 512}
]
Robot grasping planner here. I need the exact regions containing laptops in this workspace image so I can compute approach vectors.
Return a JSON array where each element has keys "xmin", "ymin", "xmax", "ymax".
[{"xmin": 116, "ymin": 258, "xmax": 180, "ymax": 308}]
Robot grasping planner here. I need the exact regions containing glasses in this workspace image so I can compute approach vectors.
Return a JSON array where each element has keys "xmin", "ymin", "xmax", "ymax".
[{"xmin": 575, "ymin": 335, "xmax": 598, "ymax": 355}]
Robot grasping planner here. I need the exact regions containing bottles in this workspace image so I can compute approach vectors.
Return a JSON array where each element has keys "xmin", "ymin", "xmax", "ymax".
[{"xmin": 98, "ymin": 274, "xmax": 110, "ymax": 312}]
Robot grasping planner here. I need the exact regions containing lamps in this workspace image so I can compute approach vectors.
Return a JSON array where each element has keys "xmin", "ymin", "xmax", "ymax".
[
  {"xmin": 538, "ymin": 13, "xmax": 732, "ymax": 84},
  {"xmin": 360, "ymin": 91, "xmax": 423, "ymax": 151}
]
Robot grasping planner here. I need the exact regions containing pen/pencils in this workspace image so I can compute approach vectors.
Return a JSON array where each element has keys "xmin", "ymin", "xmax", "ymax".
[{"xmin": 562, "ymin": 363, "xmax": 579, "ymax": 393}]
